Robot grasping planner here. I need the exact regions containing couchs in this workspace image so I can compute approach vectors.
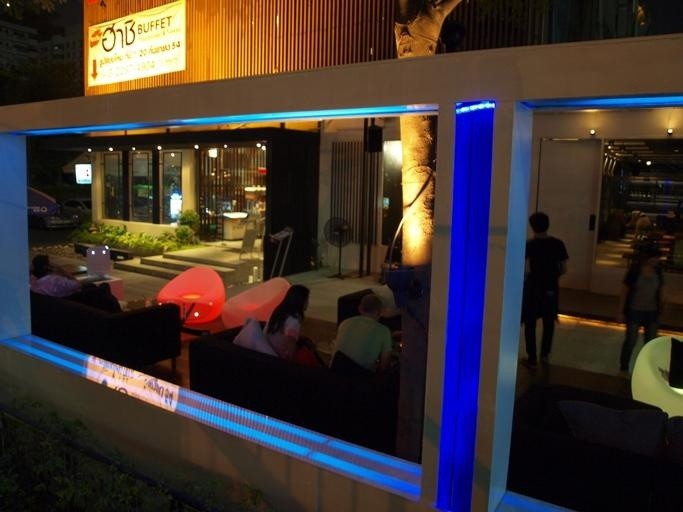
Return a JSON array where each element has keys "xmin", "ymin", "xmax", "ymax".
[
  {"xmin": 144, "ymin": 263, "xmax": 226, "ymax": 330},
  {"xmin": 187, "ymin": 321, "xmax": 388, "ymax": 446},
  {"xmin": 664, "ymin": 414, "xmax": 682, "ymax": 512},
  {"xmin": 336, "ymin": 287, "xmax": 402, "ymax": 334},
  {"xmin": 628, "ymin": 333, "xmax": 682, "ymax": 414},
  {"xmin": 28, "ymin": 269, "xmax": 182, "ymax": 373},
  {"xmin": 222, "ymin": 273, "xmax": 290, "ymax": 328},
  {"xmin": 507, "ymin": 383, "xmax": 665, "ymax": 510}
]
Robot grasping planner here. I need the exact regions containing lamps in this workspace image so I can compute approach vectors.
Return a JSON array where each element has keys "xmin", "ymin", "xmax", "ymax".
[{"xmin": 72, "ymin": 151, "xmax": 93, "ymax": 186}]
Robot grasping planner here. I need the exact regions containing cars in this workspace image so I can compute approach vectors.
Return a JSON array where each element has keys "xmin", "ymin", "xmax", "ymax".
[{"xmin": 27, "ymin": 176, "xmax": 152, "ymax": 230}]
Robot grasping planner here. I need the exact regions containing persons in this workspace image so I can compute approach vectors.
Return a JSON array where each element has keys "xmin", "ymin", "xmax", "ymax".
[
  {"xmin": 616, "ymin": 247, "xmax": 664, "ymax": 382},
  {"xmin": 399, "ymin": 307, "xmax": 427, "ymax": 386},
  {"xmin": 262, "ymin": 284, "xmax": 328, "ymax": 369},
  {"xmin": 29, "ymin": 255, "xmax": 121, "ymax": 313},
  {"xmin": 329, "ymin": 294, "xmax": 398, "ymax": 372},
  {"xmin": 633, "ymin": 211, "xmax": 653, "ymax": 243},
  {"xmin": 520, "ymin": 212, "xmax": 568, "ymax": 369}
]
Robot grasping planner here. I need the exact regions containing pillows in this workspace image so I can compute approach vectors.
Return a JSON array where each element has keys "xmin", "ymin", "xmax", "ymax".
[
  {"xmin": 326, "ymin": 351, "xmax": 371, "ymax": 387},
  {"xmin": 269, "ymin": 329, "xmax": 331, "ymax": 362},
  {"xmin": 557, "ymin": 396, "xmax": 667, "ymax": 456},
  {"xmin": 367, "ymin": 285, "xmax": 396, "ymax": 308},
  {"xmin": 233, "ymin": 320, "xmax": 277, "ymax": 359},
  {"xmin": 667, "ymin": 339, "xmax": 681, "ymax": 387},
  {"xmin": 285, "ymin": 338, "xmax": 325, "ymax": 370}
]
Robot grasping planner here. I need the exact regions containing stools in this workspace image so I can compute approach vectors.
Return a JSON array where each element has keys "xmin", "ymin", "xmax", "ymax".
[{"xmin": 623, "ymin": 215, "xmax": 676, "ymax": 269}]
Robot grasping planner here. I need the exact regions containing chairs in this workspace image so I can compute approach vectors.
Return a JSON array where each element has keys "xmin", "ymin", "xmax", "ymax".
[{"xmin": 223, "ymin": 187, "xmax": 266, "ymax": 260}]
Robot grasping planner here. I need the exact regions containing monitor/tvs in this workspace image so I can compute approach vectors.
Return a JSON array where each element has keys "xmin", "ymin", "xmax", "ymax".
[{"xmin": 86, "ymin": 245, "xmax": 112, "ymax": 281}]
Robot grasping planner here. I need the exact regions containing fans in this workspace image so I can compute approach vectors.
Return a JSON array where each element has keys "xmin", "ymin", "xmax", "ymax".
[{"xmin": 324, "ymin": 217, "xmax": 353, "ymax": 280}]
